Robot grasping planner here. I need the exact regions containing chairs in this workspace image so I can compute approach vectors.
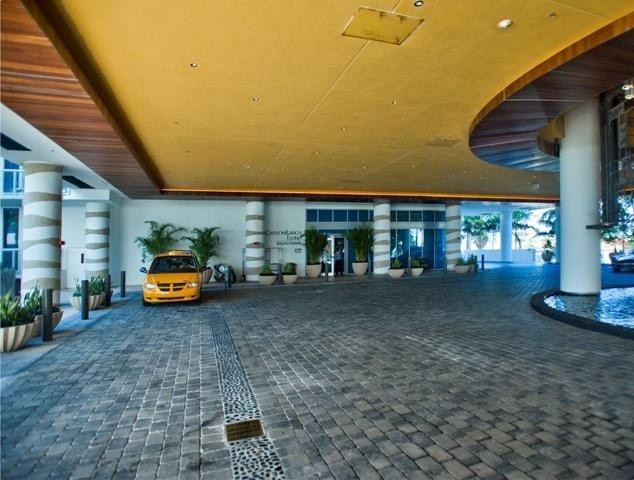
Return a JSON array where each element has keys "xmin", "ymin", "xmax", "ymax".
[{"xmin": 153, "ymin": 259, "xmax": 170, "ymax": 271}]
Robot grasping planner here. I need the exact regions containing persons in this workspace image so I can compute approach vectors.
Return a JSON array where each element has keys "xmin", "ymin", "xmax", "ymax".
[
  {"xmin": 177, "ymin": 258, "xmax": 194, "ymax": 268},
  {"xmin": 334, "ymin": 248, "xmax": 343, "ymax": 277}
]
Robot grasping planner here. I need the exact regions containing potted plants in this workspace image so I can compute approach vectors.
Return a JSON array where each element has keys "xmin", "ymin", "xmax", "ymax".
[
  {"xmin": 388, "ymin": 258, "xmax": 423, "ymax": 278},
  {"xmin": 342, "ymin": 222, "xmax": 375, "ymax": 275},
  {"xmin": 297, "ymin": 227, "xmax": 329, "ymax": 277},
  {"xmin": 259, "ymin": 263, "xmax": 297, "ymax": 285},
  {"xmin": 189, "ymin": 227, "xmax": 223, "ymax": 282},
  {"xmin": 70, "ymin": 274, "xmax": 112, "ymax": 311},
  {"xmin": 455, "ymin": 254, "xmax": 476, "ymax": 274},
  {"xmin": 0, "ymin": 281, "xmax": 64, "ymax": 352}
]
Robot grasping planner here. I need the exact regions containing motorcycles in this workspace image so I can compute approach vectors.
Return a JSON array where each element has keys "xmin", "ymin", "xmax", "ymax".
[{"xmin": 214, "ymin": 263, "xmax": 236, "ymax": 283}]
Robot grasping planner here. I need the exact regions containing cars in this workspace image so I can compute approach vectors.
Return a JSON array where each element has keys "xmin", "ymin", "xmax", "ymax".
[
  {"xmin": 141, "ymin": 250, "xmax": 214, "ymax": 306},
  {"xmin": 612, "ymin": 248, "xmax": 634, "ymax": 270}
]
